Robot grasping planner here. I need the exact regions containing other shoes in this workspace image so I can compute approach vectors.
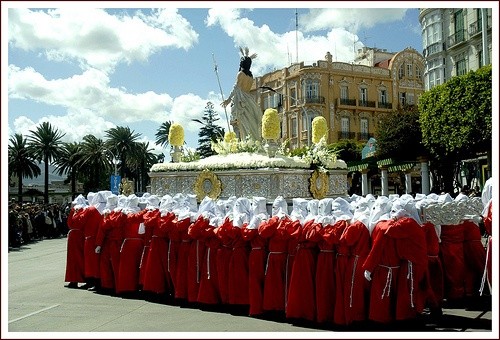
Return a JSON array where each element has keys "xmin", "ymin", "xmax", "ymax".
[{"xmin": 65, "ymin": 280, "xmax": 251, "ymax": 315}]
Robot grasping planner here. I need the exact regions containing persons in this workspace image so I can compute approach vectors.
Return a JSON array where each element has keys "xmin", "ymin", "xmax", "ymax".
[
  {"xmin": 9, "ymin": 176, "xmax": 493, "ymax": 334},
  {"xmin": 220, "ymin": 56, "xmax": 264, "ymax": 143}
]
